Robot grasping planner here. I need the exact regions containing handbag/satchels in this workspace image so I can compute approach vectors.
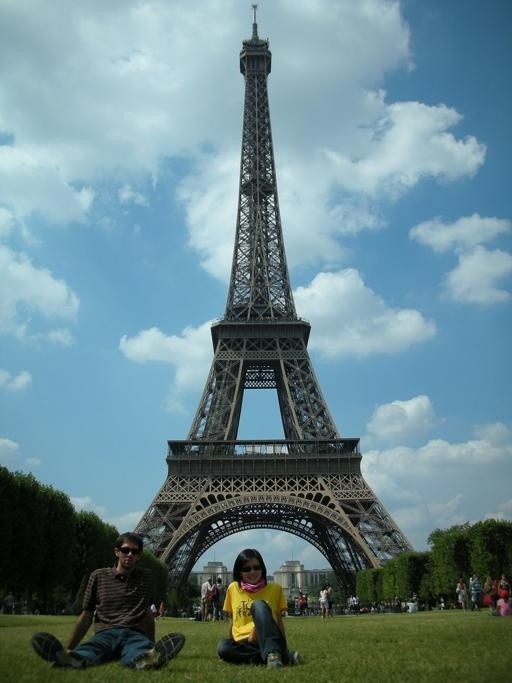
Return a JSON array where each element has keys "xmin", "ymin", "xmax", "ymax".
[{"xmin": 206, "ymin": 590, "xmax": 215, "ymax": 602}]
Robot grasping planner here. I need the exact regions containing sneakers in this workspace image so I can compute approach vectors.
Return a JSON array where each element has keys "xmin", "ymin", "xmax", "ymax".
[
  {"xmin": 289, "ymin": 651, "xmax": 302, "ymax": 666},
  {"xmin": 31, "ymin": 632, "xmax": 87, "ymax": 670},
  {"xmin": 133, "ymin": 632, "xmax": 186, "ymax": 671},
  {"xmin": 265, "ymin": 650, "xmax": 283, "ymax": 671}
]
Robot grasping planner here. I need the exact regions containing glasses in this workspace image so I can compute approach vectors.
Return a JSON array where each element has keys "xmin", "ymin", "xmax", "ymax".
[
  {"xmin": 242, "ymin": 564, "xmax": 262, "ymax": 573},
  {"xmin": 118, "ymin": 547, "xmax": 141, "ymax": 556}
]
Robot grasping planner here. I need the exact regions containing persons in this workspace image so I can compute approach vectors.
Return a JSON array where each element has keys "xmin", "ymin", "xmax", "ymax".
[
  {"xmin": 30, "ymin": 531, "xmax": 186, "ymax": 672},
  {"xmin": 4, "ymin": 589, "xmax": 17, "ymax": 613},
  {"xmin": 312, "ymin": 594, "xmax": 384, "ymax": 616},
  {"xmin": 297, "ymin": 590, "xmax": 307, "ymax": 615},
  {"xmin": 405, "ymin": 598, "xmax": 418, "ymax": 613},
  {"xmin": 324, "ymin": 582, "xmax": 335, "ymax": 618},
  {"xmin": 486, "ymin": 589, "xmax": 512, "ymax": 615},
  {"xmin": 154, "ymin": 598, "xmax": 168, "ymax": 621},
  {"xmin": 318, "ymin": 586, "xmax": 330, "ymax": 618},
  {"xmin": 453, "ymin": 570, "xmax": 512, "ymax": 611},
  {"xmin": 192, "ymin": 575, "xmax": 232, "ymax": 622},
  {"xmin": 216, "ymin": 547, "xmax": 302, "ymax": 672}
]
